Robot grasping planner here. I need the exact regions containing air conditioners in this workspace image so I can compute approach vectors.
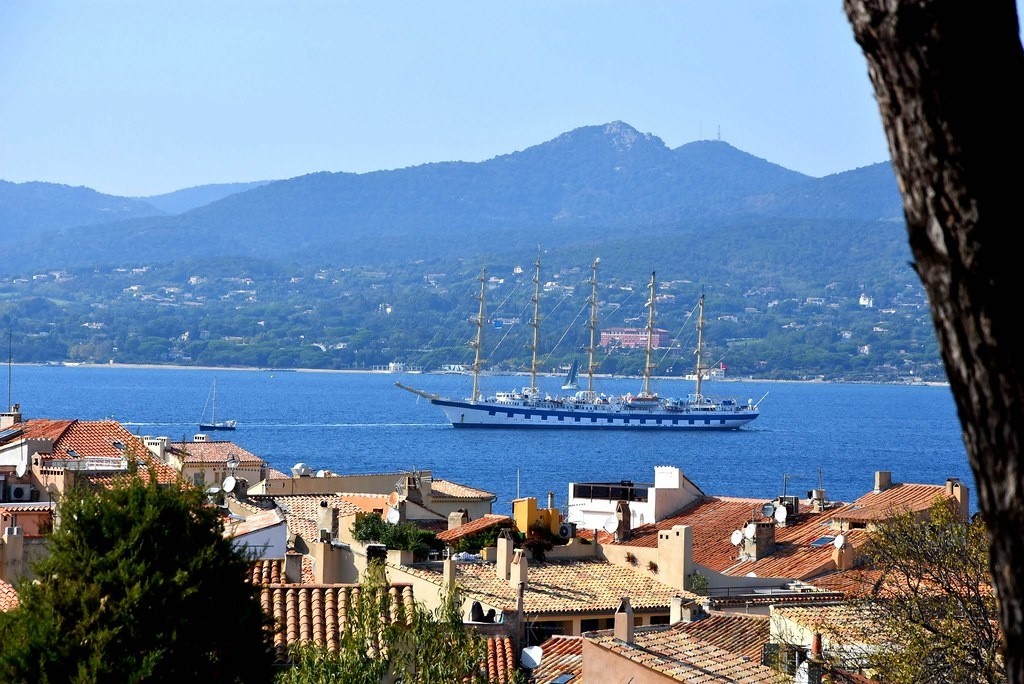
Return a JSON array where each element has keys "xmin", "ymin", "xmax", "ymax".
[
  {"xmin": 11, "ymin": 484, "xmax": 31, "ymax": 500},
  {"xmin": 558, "ymin": 523, "xmax": 573, "ymax": 538}
]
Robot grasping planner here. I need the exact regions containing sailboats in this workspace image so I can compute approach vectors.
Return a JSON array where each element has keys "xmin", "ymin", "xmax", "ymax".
[
  {"xmin": 196, "ymin": 377, "xmax": 239, "ymax": 432},
  {"xmin": 390, "ymin": 248, "xmax": 767, "ymax": 433}
]
